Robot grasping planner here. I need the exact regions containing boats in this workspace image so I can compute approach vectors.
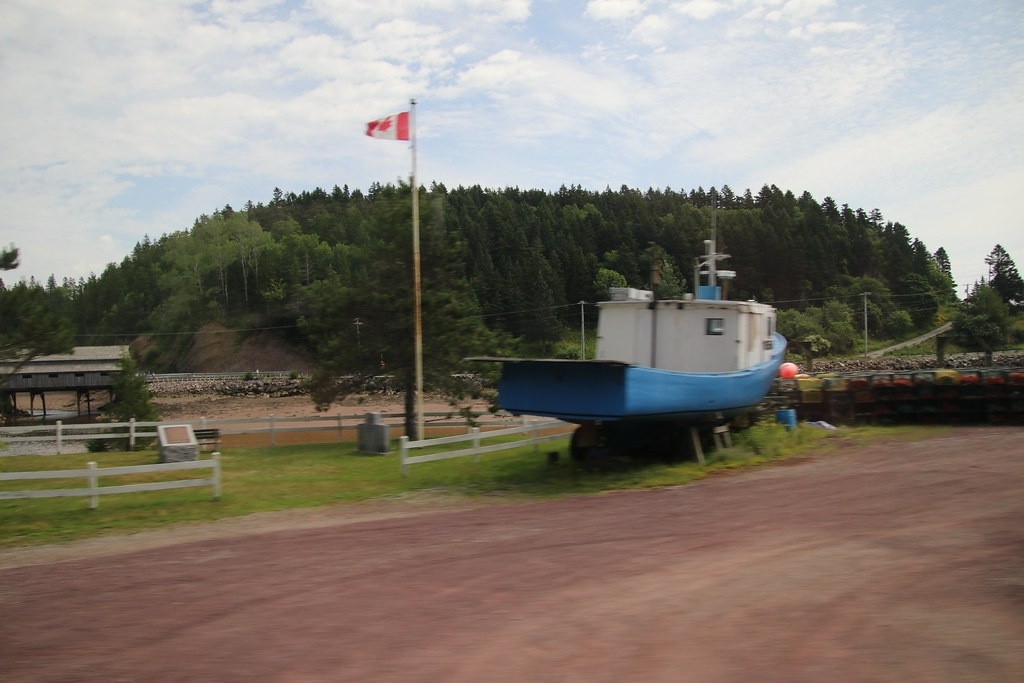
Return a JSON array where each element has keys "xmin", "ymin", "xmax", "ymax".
[{"xmin": 459, "ymin": 239, "xmax": 789, "ymax": 417}]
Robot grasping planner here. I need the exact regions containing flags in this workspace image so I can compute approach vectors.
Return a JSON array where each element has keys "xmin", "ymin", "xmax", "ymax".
[{"xmin": 366, "ymin": 112, "xmax": 410, "ymax": 140}]
